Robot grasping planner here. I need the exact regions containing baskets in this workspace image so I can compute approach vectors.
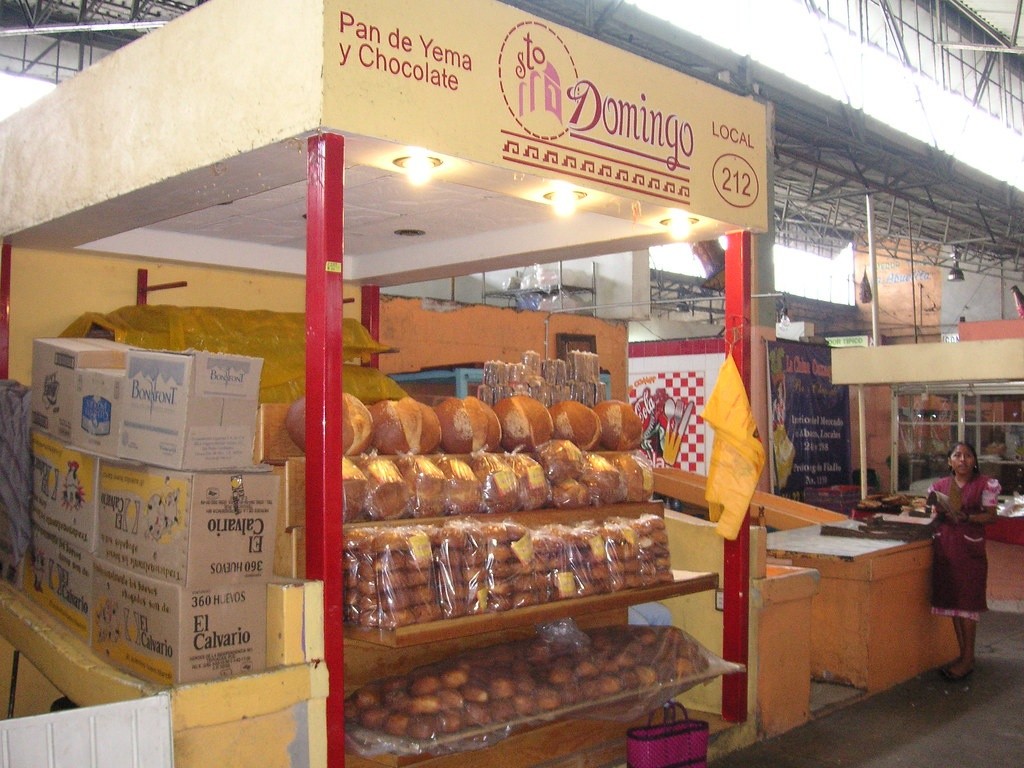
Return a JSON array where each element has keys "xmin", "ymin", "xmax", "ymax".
[{"xmin": 626, "ymin": 701, "xmax": 709, "ymax": 768}]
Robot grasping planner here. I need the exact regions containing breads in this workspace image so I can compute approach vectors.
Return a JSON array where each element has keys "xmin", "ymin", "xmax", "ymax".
[
  {"xmin": 343, "ymin": 621, "xmax": 709, "ymax": 740},
  {"xmin": 860, "ymin": 494, "xmax": 903, "ymax": 507},
  {"xmin": 342, "ymin": 511, "xmax": 675, "ymax": 632},
  {"xmin": 285, "ymin": 392, "xmax": 654, "ymax": 521}
]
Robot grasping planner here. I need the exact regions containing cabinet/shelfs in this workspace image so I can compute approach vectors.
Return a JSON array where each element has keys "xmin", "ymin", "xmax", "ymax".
[
  {"xmin": 886, "ymin": 404, "xmax": 1024, "ymax": 499},
  {"xmin": 283, "ymin": 452, "xmax": 746, "ymax": 767}
]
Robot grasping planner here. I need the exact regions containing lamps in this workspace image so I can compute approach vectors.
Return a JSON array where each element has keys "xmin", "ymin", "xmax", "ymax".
[
  {"xmin": 780, "ymin": 308, "xmax": 791, "ymax": 327},
  {"xmin": 947, "ymin": 260, "xmax": 965, "ymax": 282}
]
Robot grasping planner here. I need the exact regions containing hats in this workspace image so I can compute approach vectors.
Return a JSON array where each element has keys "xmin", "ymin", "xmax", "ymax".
[{"xmin": 981, "ymin": 428, "xmax": 1005, "ymax": 447}]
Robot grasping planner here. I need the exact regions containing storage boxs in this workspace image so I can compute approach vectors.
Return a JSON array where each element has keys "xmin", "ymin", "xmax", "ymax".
[
  {"xmin": 777, "ymin": 322, "xmax": 814, "ymax": 342},
  {"xmin": 71, "ymin": 367, "xmax": 125, "ymax": 458},
  {"xmin": 116, "ymin": 350, "xmax": 265, "ymax": 470},
  {"xmin": 626, "ymin": 700, "xmax": 709, "ymax": 768},
  {"xmin": 28, "ymin": 428, "xmax": 103, "ymax": 580},
  {"xmin": 22, "ymin": 521, "xmax": 96, "ymax": 657},
  {"xmin": 91, "ymin": 554, "xmax": 267, "ymax": 688},
  {"xmin": 30, "ymin": 337, "xmax": 144, "ymax": 446},
  {"xmin": 95, "ymin": 454, "xmax": 282, "ymax": 588}
]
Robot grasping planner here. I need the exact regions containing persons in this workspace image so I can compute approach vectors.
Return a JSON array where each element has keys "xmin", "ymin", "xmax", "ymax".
[{"xmin": 929, "ymin": 441, "xmax": 1002, "ymax": 682}]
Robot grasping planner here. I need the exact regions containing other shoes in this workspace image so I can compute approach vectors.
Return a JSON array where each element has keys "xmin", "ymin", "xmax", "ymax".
[{"xmin": 937, "ymin": 657, "xmax": 972, "ymax": 683}]
